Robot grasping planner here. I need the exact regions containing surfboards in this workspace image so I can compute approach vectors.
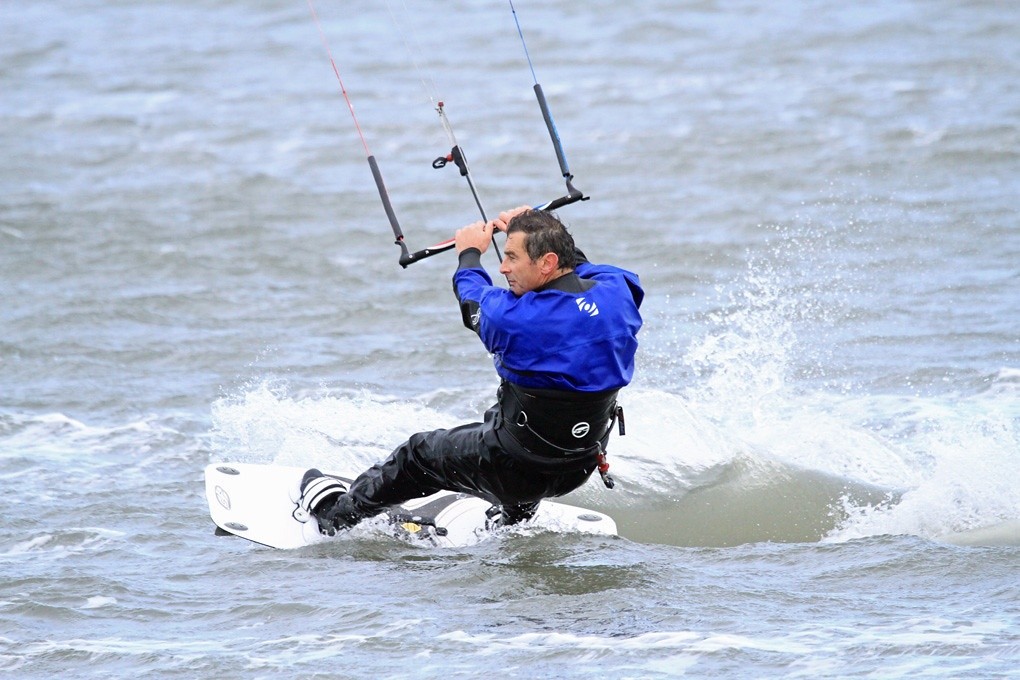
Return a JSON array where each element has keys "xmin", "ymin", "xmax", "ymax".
[{"xmin": 203, "ymin": 461, "xmax": 618, "ymax": 549}]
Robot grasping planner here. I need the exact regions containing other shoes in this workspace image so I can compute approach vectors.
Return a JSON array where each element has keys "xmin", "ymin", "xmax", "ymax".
[{"xmin": 300, "ymin": 468, "xmax": 351, "ymax": 536}]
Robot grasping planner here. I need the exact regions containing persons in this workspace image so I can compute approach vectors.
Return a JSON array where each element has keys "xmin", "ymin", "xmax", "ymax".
[{"xmin": 300, "ymin": 206, "xmax": 645, "ymax": 536}]
